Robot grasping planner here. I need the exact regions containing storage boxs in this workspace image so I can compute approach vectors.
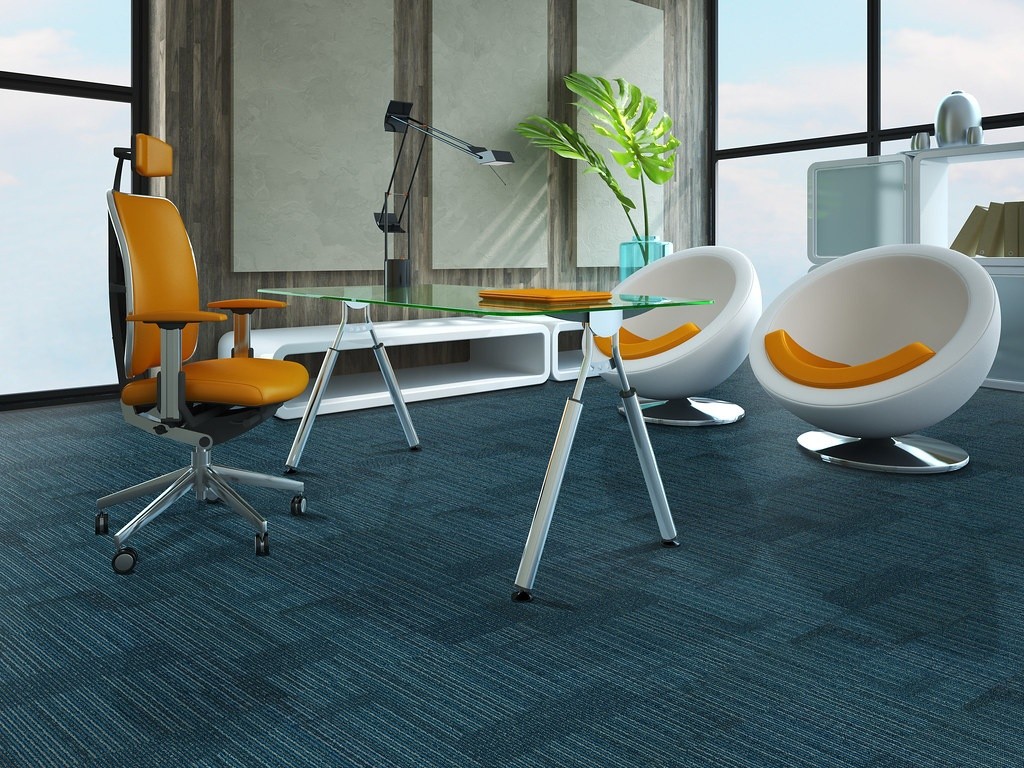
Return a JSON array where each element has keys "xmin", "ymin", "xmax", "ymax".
[
  {"xmin": 1018, "ymin": 201, "xmax": 1024, "ymax": 257},
  {"xmin": 949, "ymin": 205, "xmax": 989, "ymax": 257},
  {"xmin": 975, "ymin": 202, "xmax": 1004, "ymax": 257},
  {"xmin": 1004, "ymin": 202, "xmax": 1018, "ymax": 257}
]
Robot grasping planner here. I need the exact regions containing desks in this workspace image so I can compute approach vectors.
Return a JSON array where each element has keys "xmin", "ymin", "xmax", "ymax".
[
  {"xmin": 258, "ymin": 285, "xmax": 716, "ymax": 604},
  {"xmin": 216, "ymin": 316, "xmax": 551, "ymax": 420},
  {"xmin": 482, "ymin": 315, "xmax": 601, "ymax": 381}
]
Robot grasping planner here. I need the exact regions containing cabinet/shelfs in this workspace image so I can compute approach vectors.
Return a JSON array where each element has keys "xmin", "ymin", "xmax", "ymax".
[
  {"xmin": 979, "ymin": 275, "xmax": 1024, "ymax": 393},
  {"xmin": 807, "ymin": 153, "xmax": 912, "ymax": 263},
  {"xmin": 912, "ymin": 141, "xmax": 1024, "ymax": 266}
]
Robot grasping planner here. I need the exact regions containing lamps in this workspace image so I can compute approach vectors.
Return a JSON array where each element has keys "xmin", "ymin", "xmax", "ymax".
[{"xmin": 373, "ymin": 100, "xmax": 515, "ymax": 286}]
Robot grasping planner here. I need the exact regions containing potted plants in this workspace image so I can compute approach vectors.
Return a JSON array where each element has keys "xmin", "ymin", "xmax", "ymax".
[{"xmin": 513, "ymin": 73, "xmax": 681, "ymax": 283}]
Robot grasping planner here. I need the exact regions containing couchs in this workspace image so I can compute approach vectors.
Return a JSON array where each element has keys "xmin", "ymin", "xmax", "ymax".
[
  {"xmin": 749, "ymin": 243, "xmax": 1003, "ymax": 475},
  {"xmin": 580, "ymin": 246, "xmax": 762, "ymax": 427}
]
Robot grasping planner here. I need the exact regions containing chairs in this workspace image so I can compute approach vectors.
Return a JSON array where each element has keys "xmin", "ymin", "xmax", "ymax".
[{"xmin": 94, "ymin": 133, "xmax": 310, "ymax": 575}]
